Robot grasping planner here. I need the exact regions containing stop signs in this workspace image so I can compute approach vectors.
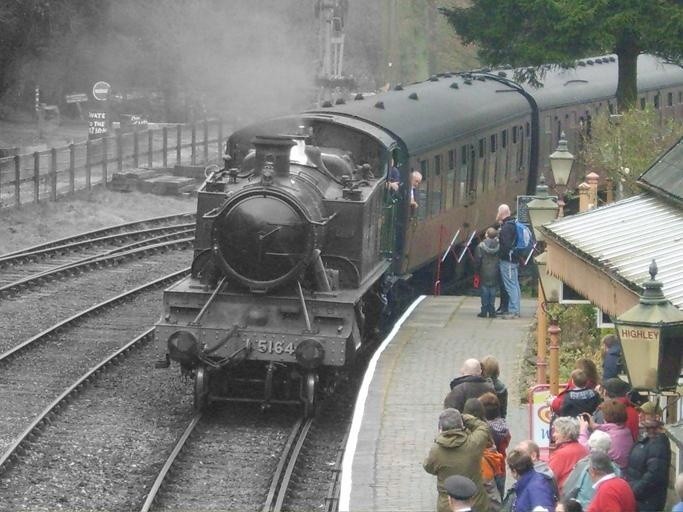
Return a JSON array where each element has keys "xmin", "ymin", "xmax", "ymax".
[{"xmin": 90, "ymin": 80, "xmax": 111, "ymax": 102}]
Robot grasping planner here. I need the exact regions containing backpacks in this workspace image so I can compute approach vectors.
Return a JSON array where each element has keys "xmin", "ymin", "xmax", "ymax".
[{"xmin": 507, "ymin": 220, "xmax": 532, "ymax": 257}]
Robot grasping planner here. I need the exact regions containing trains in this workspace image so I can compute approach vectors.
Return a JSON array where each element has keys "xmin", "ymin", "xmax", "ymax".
[{"xmin": 147, "ymin": 51, "xmax": 683, "ymax": 420}]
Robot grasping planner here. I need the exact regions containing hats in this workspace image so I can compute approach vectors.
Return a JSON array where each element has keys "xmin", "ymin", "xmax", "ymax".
[
  {"xmin": 635, "ymin": 401, "xmax": 664, "ymax": 415},
  {"xmin": 444, "ymin": 475, "xmax": 476, "ymax": 499}
]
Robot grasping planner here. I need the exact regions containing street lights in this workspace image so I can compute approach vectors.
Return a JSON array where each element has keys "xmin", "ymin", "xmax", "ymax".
[
  {"xmin": 524, "ymin": 127, "xmax": 576, "ymax": 413},
  {"xmin": 612, "ymin": 257, "xmax": 681, "ymax": 450}
]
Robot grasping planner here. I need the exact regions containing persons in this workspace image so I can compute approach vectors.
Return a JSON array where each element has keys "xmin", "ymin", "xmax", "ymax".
[
  {"xmin": 387, "ymin": 157, "xmax": 423, "ymax": 257},
  {"xmin": 473, "ymin": 203, "xmax": 521, "ymax": 321},
  {"xmin": 424, "ymin": 334, "xmax": 683, "ymax": 512}
]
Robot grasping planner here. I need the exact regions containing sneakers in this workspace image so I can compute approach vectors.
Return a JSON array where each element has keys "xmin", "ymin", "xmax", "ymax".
[{"xmin": 477, "ymin": 308, "xmax": 519, "ymax": 319}]
